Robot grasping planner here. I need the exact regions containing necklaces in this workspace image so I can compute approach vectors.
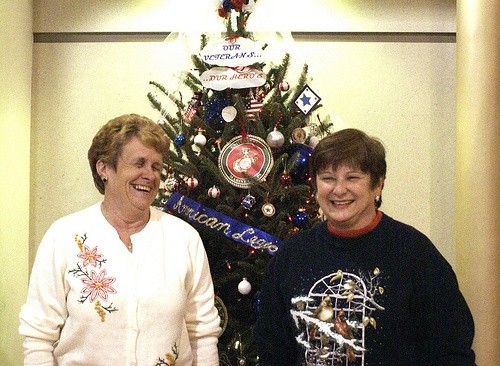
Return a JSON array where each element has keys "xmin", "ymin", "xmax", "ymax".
[{"xmin": 127, "ymin": 244, "xmax": 132, "ymax": 252}]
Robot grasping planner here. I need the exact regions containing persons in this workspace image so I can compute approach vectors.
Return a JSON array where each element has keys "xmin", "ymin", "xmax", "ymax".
[
  {"xmin": 19, "ymin": 114, "xmax": 222, "ymax": 366},
  {"xmin": 252, "ymin": 128, "xmax": 475, "ymax": 366}
]
